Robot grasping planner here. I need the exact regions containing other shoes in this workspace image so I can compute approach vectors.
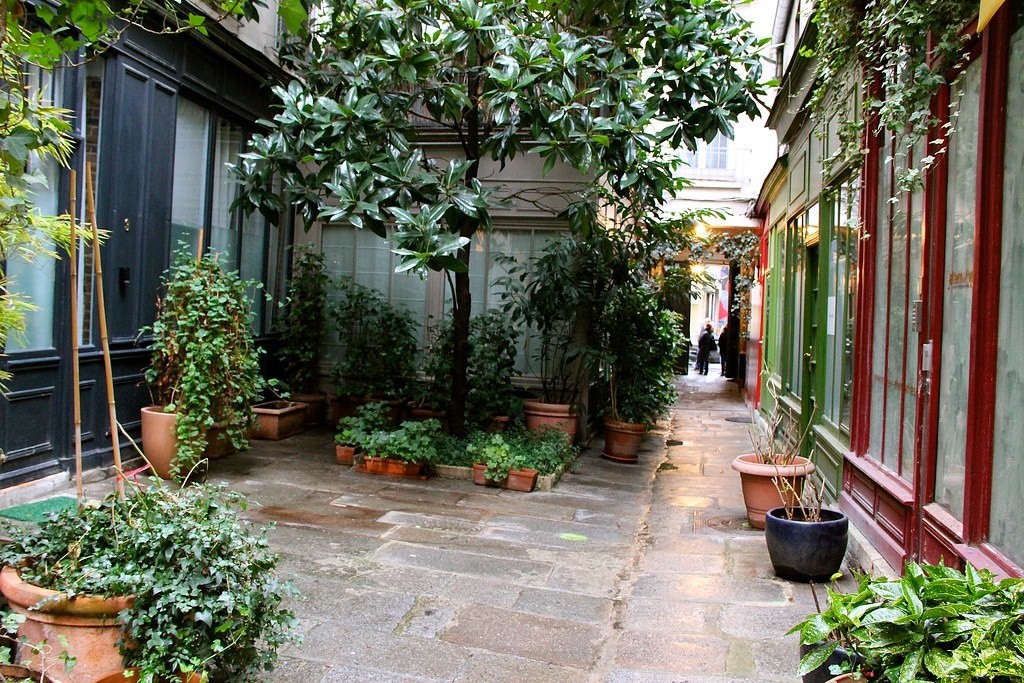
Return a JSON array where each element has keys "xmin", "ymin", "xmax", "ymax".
[
  {"xmin": 699, "ymin": 373, "xmax": 703, "ymax": 375},
  {"xmin": 704, "ymin": 373, "xmax": 707, "ymax": 375}
]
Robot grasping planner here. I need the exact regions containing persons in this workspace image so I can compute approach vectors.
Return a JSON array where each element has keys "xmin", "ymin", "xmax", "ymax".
[{"xmin": 693, "ymin": 318, "xmax": 728, "ymax": 377}]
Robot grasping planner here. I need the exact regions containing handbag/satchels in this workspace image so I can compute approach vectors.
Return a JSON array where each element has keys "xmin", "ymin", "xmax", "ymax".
[{"xmin": 711, "ymin": 335, "xmax": 717, "ymax": 351}]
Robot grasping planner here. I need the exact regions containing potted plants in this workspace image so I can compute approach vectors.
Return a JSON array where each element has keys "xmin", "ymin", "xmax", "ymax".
[
  {"xmin": 0, "ymin": 238, "xmax": 589, "ymax": 683},
  {"xmin": 793, "ymin": 578, "xmax": 867, "ymax": 683},
  {"xmin": 765, "ymin": 453, "xmax": 847, "ymax": 573},
  {"xmin": 597, "ymin": 287, "xmax": 668, "ymax": 464},
  {"xmin": 798, "ymin": 558, "xmax": 1024, "ymax": 683},
  {"xmin": 732, "ymin": 351, "xmax": 818, "ymax": 530}
]
